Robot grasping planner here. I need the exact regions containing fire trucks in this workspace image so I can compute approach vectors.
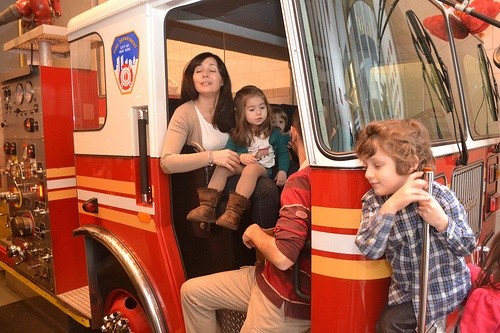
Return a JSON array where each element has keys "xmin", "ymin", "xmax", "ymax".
[{"xmin": 1, "ymin": 0, "xmax": 500, "ymax": 333}]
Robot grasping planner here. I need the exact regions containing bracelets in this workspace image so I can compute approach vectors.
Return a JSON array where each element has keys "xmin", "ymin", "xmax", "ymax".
[{"xmin": 208, "ymin": 150, "xmax": 215, "ymax": 166}]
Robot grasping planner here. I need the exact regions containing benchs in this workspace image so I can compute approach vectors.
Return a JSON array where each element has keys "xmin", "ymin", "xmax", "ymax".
[{"xmin": 167, "ymin": 98, "xmax": 297, "ymax": 273}]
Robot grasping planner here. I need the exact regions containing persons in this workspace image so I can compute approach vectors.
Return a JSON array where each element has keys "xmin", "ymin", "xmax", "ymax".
[
  {"xmin": 160, "ymin": 53, "xmax": 300, "ymax": 266},
  {"xmin": 354, "ymin": 119, "xmax": 477, "ymax": 333},
  {"xmin": 271, "ymin": 109, "xmax": 288, "ymax": 132},
  {"xmin": 446, "ymin": 231, "xmax": 500, "ymax": 333},
  {"xmin": 180, "ymin": 108, "xmax": 311, "ymax": 333},
  {"xmin": 186, "ymin": 86, "xmax": 290, "ymax": 231}
]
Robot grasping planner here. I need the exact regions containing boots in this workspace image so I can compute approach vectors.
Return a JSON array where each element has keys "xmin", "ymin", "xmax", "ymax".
[
  {"xmin": 216, "ymin": 192, "xmax": 250, "ymax": 230},
  {"xmin": 187, "ymin": 187, "xmax": 220, "ymax": 223}
]
